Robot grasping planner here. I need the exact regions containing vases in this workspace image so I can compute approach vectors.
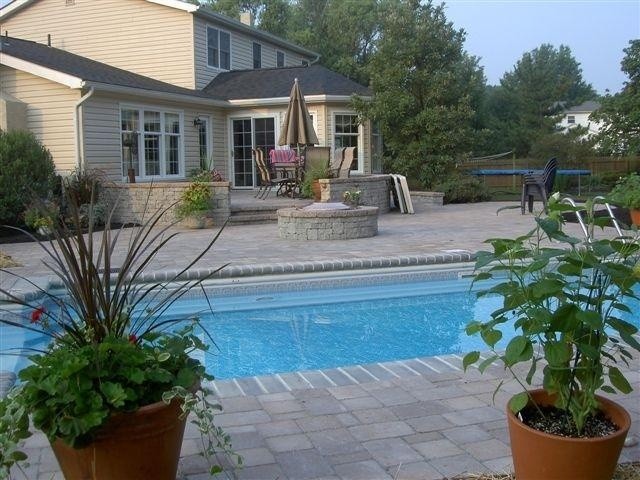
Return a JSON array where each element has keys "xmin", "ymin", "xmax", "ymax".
[{"xmin": 35, "ymin": 383, "xmax": 195, "ymax": 480}]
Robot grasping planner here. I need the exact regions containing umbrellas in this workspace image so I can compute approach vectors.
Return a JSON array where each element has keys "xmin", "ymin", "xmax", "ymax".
[{"xmin": 277, "ymin": 76, "xmax": 320, "ymax": 191}]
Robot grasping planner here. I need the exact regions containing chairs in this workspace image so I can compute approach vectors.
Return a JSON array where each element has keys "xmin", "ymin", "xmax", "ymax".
[
  {"xmin": 520, "ymin": 157, "xmax": 556, "ymax": 215},
  {"xmin": 252, "ymin": 147, "xmax": 355, "ymax": 203}
]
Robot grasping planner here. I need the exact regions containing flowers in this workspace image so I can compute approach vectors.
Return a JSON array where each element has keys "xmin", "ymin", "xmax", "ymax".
[{"xmin": 1, "ymin": 178, "xmax": 248, "ymax": 427}]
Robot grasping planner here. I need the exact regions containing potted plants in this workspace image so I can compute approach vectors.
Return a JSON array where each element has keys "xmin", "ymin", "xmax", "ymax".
[
  {"xmin": 22, "ymin": 198, "xmax": 62, "ymax": 238},
  {"xmin": 169, "ymin": 184, "xmax": 217, "ymax": 231}
]
